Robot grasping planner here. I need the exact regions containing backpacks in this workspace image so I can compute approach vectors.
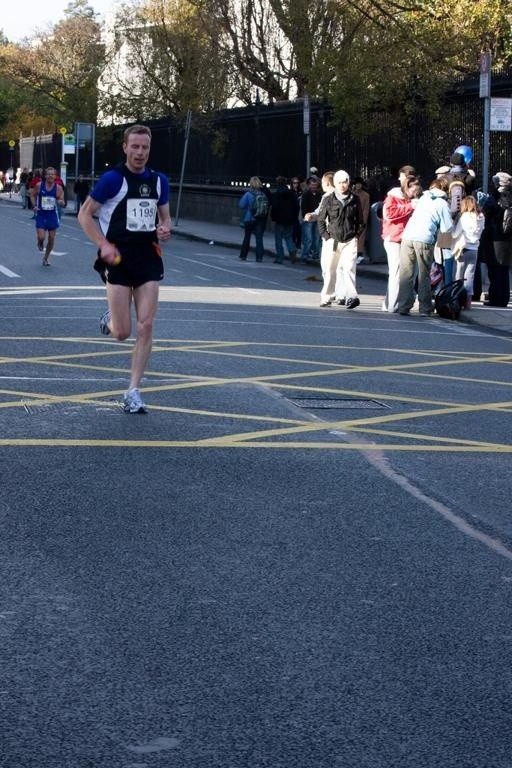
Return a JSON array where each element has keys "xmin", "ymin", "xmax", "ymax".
[
  {"xmin": 435, "ymin": 278, "xmax": 467, "ymax": 319},
  {"xmin": 248, "ymin": 191, "xmax": 268, "ymax": 218}
]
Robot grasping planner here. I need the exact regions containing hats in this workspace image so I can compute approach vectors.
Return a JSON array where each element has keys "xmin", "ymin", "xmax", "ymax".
[
  {"xmin": 450, "ymin": 154, "xmax": 465, "ymax": 167},
  {"xmin": 434, "ymin": 166, "xmax": 451, "ymax": 175}
]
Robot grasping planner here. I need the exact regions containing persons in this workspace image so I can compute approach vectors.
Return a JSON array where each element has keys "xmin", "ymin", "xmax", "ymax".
[
  {"xmin": 310, "ymin": 167, "xmax": 320, "ymax": 181},
  {"xmin": 77, "ymin": 124, "xmax": 172, "ymax": 414},
  {"xmin": 238, "ymin": 176, "xmax": 270, "ymax": 262},
  {"xmin": 30, "ymin": 167, "xmax": 65, "ymax": 267},
  {"xmin": 479, "ymin": 171, "xmax": 512, "ymax": 307},
  {"xmin": 1, "ymin": 167, "xmax": 30, "ymax": 212},
  {"xmin": 397, "ymin": 165, "xmax": 416, "ymax": 185},
  {"xmin": 317, "ymin": 170, "xmax": 363, "ymax": 310},
  {"xmin": 301, "ymin": 176, "xmax": 321, "ymax": 265},
  {"xmin": 351, "ymin": 177, "xmax": 370, "ymax": 266},
  {"xmin": 290, "ymin": 176, "xmax": 301, "ymax": 197},
  {"xmin": 303, "ymin": 171, "xmax": 336, "ymax": 222},
  {"xmin": 262, "ymin": 175, "xmax": 300, "ymax": 264},
  {"xmin": 382, "ymin": 175, "xmax": 422, "ymax": 313},
  {"xmin": 433, "ymin": 153, "xmax": 479, "ymax": 300},
  {"xmin": 74, "ymin": 174, "xmax": 88, "ymax": 218},
  {"xmin": 435, "ymin": 165, "xmax": 452, "ymax": 178},
  {"xmin": 452, "ymin": 195, "xmax": 485, "ymax": 310},
  {"xmin": 400, "ymin": 179, "xmax": 455, "ymax": 316}
]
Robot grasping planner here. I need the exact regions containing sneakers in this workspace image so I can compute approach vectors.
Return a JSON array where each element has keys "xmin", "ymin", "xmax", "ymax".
[
  {"xmin": 124, "ymin": 389, "xmax": 147, "ymax": 413},
  {"xmin": 346, "ymin": 299, "xmax": 359, "ymax": 309},
  {"xmin": 99, "ymin": 310, "xmax": 111, "ymax": 334}
]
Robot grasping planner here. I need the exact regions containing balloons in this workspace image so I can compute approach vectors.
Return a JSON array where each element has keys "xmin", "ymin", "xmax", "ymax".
[{"xmin": 454, "ymin": 145, "xmax": 473, "ymax": 164}]
